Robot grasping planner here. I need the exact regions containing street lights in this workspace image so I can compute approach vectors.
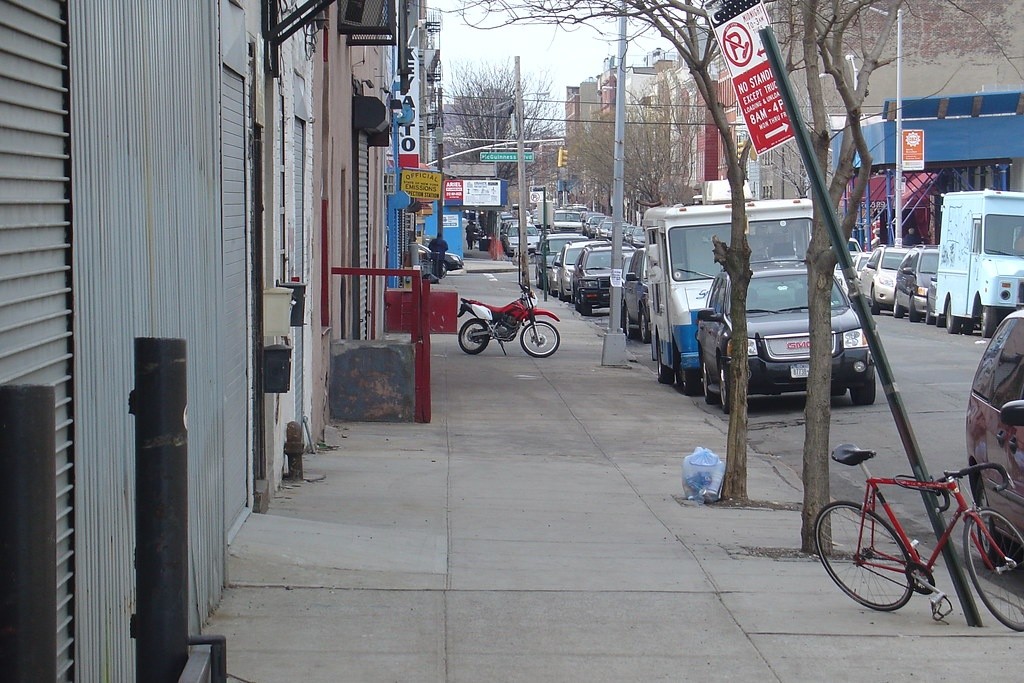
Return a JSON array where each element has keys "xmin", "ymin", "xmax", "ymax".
[
  {"xmin": 602, "ymin": 85, "xmax": 646, "ymax": 164},
  {"xmin": 492, "ymin": 90, "xmax": 550, "ymax": 180}
]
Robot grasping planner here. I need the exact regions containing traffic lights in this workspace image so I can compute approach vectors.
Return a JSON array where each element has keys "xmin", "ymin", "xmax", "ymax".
[{"xmin": 557, "ymin": 146, "xmax": 568, "ymax": 168}]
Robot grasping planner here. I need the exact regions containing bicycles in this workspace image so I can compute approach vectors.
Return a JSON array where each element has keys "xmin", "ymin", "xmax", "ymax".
[{"xmin": 812, "ymin": 443, "xmax": 1024, "ymax": 633}]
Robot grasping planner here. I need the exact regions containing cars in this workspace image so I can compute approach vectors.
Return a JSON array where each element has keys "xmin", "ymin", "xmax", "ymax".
[
  {"xmin": 497, "ymin": 198, "xmax": 940, "ymax": 414},
  {"xmin": 964, "ymin": 307, "xmax": 1024, "ymax": 573}
]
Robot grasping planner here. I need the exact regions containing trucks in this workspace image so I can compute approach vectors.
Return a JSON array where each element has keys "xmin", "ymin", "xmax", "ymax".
[{"xmin": 932, "ymin": 188, "xmax": 1024, "ymax": 339}]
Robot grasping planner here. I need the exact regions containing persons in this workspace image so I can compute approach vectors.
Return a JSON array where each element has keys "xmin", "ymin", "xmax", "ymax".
[
  {"xmin": 429, "ymin": 233, "xmax": 448, "ymax": 279},
  {"xmin": 466, "ymin": 221, "xmax": 476, "ymax": 250},
  {"xmin": 902, "ymin": 227, "xmax": 924, "ymax": 246}
]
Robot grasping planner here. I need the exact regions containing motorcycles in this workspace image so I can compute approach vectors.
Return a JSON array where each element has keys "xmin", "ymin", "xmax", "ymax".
[{"xmin": 457, "ymin": 271, "xmax": 561, "ymax": 358}]
